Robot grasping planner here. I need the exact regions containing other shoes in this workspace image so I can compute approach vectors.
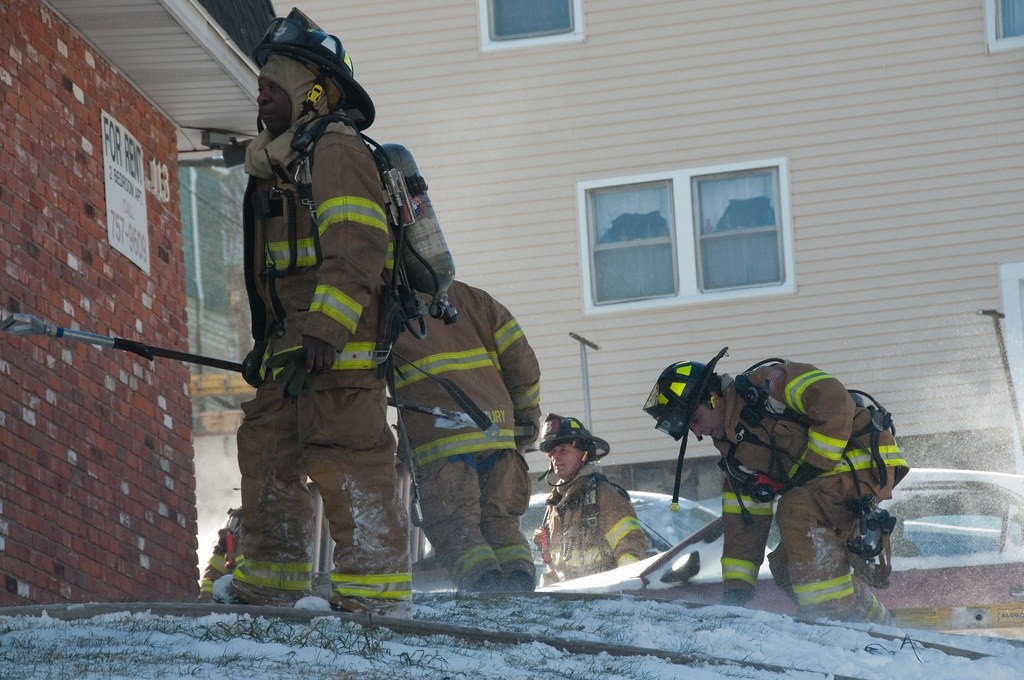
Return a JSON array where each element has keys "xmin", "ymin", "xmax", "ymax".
[
  {"xmin": 470, "ymin": 569, "xmax": 535, "ymax": 593},
  {"xmin": 643, "ymin": 346, "xmax": 729, "ymax": 441},
  {"xmin": 211, "ymin": 572, "xmax": 253, "ymax": 605}
]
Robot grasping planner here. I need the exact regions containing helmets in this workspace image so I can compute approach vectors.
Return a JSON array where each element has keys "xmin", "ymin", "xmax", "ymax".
[
  {"xmin": 253, "ymin": 7, "xmax": 375, "ymax": 132},
  {"xmin": 540, "ymin": 413, "xmax": 610, "ymax": 462}
]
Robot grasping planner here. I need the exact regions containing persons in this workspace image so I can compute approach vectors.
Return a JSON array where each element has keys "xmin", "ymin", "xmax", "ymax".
[
  {"xmin": 643, "ymin": 347, "xmax": 909, "ymax": 625},
  {"xmin": 388, "ymin": 281, "xmax": 542, "ymax": 591},
  {"xmin": 533, "ymin": 413, "xmax": 645, "ymax": 585},
  {"xmin": 232, "ymin": 6, "xmax": 428, "ymax": 618},
  {"xmin": 199, "ymin": 506, "xmax": 243, "ymax": 602}
]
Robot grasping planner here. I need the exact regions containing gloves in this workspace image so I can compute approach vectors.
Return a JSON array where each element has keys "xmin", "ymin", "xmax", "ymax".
[
  {"xmin": 514, "ymin": 405, "xmax": 542, "ymax": 446},
  {"xmin": 788, "ymin": 462, "xmax": 827, "ymax": 487},
  {"xmin": 265, "ymin": 346, "xmax": 319, "ymax": 402}
]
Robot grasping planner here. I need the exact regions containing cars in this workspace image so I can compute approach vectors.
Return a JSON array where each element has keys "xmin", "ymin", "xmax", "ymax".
[
  {"xmin": 705, "ymin": 468, "xmax": 1023, "ymax": 636},
  {"xmin": 478, "ymin": 488, "xmax": 777, "ymax": 591}
]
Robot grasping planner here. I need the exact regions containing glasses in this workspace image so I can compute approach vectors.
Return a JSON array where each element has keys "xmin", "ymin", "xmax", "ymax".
[{"xmin": 256, "ymin": 17, "xmax": 317, "ymax": 53}]
